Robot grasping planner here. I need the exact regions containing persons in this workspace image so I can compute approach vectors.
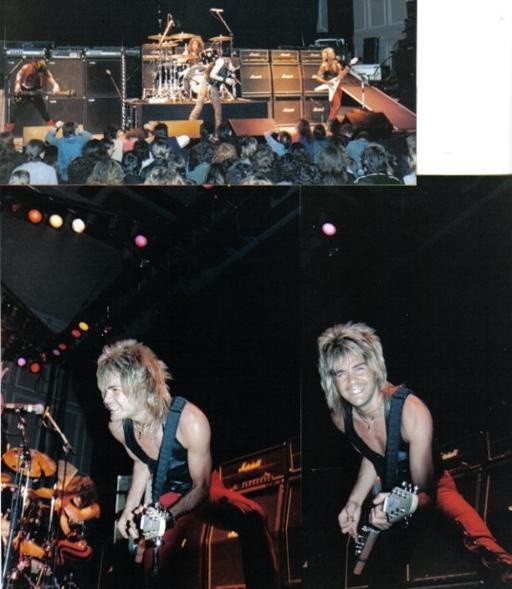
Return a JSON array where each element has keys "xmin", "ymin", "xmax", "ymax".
[
  {"xmin": 5, "ymin": 56, "xmax": 60, "ymax": 132},
  {"xmin": 95, "ymin": 339, "xmax": 280, "ymax": 588},
  {"xmin": 182, "ymin": 36, "xmax": 204, "ymax": 58},
  {"xmin": 49, "ymin": 473, "xmax": 101, "ymax": 566},
  {"xmin": 189, "ymin": 56, "xmax": 242, "ymax": 129},
  {"xmin": 316, "ymin": 47, "xmax": 350, "ymax": 125},
  {"xmin": 316, "ymin": 322, "xmax": 512, "ymax": 589},
  {"xmin": 1, "ymin": 112, "xmax": 416, "ymax": 187}
]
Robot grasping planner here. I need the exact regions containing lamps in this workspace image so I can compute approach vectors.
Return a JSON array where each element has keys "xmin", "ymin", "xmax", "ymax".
[
  {"xmin": 134, "ymin": 226, "xmax": 156, "ymax": 248},
  {"xmin": 10, "ymin": 195, "xmax": 91, "ymax": 236},
  {"xmin": 14, "ymin": 313, "xmax": 100, "ymax": 374},
  {"xmin": 320, "ymin": 200, "xmax": 342, "ymax": 238}
]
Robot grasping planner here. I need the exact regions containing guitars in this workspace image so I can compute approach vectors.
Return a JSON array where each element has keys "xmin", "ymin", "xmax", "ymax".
[
  {"xmin": 127, "ymin": 479, "xmax": 170, "ymax": 564},
  {"xmin": 56, "ymin": 499, "xmax": 71, "ymax": 536},
  {"xmin": 314, "ymin": 57, "xmax": 359, "ymax": 104},
  {"xmin": 343, "ymin": 478, "xmax": 419, "ymax": 574}
]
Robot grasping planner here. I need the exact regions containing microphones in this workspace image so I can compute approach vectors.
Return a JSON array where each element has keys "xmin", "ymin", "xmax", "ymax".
[
  {"xmin": 2, "ymin": 403, "xmax": 44, "ymax": 415},
  {"xmin": 211, "ymin": 8, "xmax": 222, "ymax": 12}
]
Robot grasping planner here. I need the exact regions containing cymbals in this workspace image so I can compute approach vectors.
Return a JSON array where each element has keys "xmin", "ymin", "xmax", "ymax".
[
  {"xmin": 35, "ymin": 488, "xmax": 57, "ymax": 499},
  {"xmin": 208, "ymin": 36, "xmax": 232, "ymax": 41},
  {"xmin": 170, "ymin": 54, "xmax": 197, "ymax": 59},
  {"xmin": 150, "ymin": 41, "xmax": 175, "ymax": 49},
  {"xmin": 2, "ymin": 448, "xmax": 56, "ymax": 479},
  {"xmin": 172, "ymin": 32, "xmax": 195, "ymax": 39},
  {"xmin": 148, "ymin": 35, "xmax": 172, "ymax": 41}
]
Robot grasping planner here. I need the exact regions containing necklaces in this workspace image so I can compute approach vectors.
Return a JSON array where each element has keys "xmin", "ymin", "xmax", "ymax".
[
  {"xmin": 358, "ymin": 407, "xmax": 380, "ymax": 432},
  {"xmin": 134, "ymin": 423, "xmax": 152, "ymax": 440}
]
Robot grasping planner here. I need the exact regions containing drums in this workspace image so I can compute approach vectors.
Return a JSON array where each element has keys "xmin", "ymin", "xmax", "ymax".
[
  {"xmin": 27, "ymin": 548, "xmax": 57, "ymax": 575},
  {"xmin": 1, "ymin": 483, "xmax": 34, "ymax": 521},
  {"xmin": 178, "ymin": 65, "xmax": 212, "ymax": 100},
  {"xmin": 201, "ymin": 48, "xmax": 218, "ymax": 64},
  {"xmin": 158, "ymin": 63, "xmax": 188, "ymax": 85},
  {"xmin": 14, "ymin": 504, "xmax": 61, "ymax": 560}
]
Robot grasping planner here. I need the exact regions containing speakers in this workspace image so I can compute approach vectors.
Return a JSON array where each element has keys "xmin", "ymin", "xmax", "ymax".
[
  {"xmin": 148, "ymin": 120, "xmax": 203, "ymax": 150},
  {"xmin": 343, "ymin": 112, "xmax": 394, "ymax": 132},
  {"xmin": 226, "ymin": 118, "xmax": 281, "ymax": 145},
  {"xmin": 23, "ymin": 124, "xmax": 84, "ymax": 154},
  {"xmin": 330, "ymin": 116, "xmax": 346, "ymax": 135}
]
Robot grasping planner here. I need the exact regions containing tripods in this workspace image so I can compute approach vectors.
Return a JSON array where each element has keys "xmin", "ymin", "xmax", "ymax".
[{"xmin": 144, "ymin": 13, "xmax": 251, "ymax": 104}]
